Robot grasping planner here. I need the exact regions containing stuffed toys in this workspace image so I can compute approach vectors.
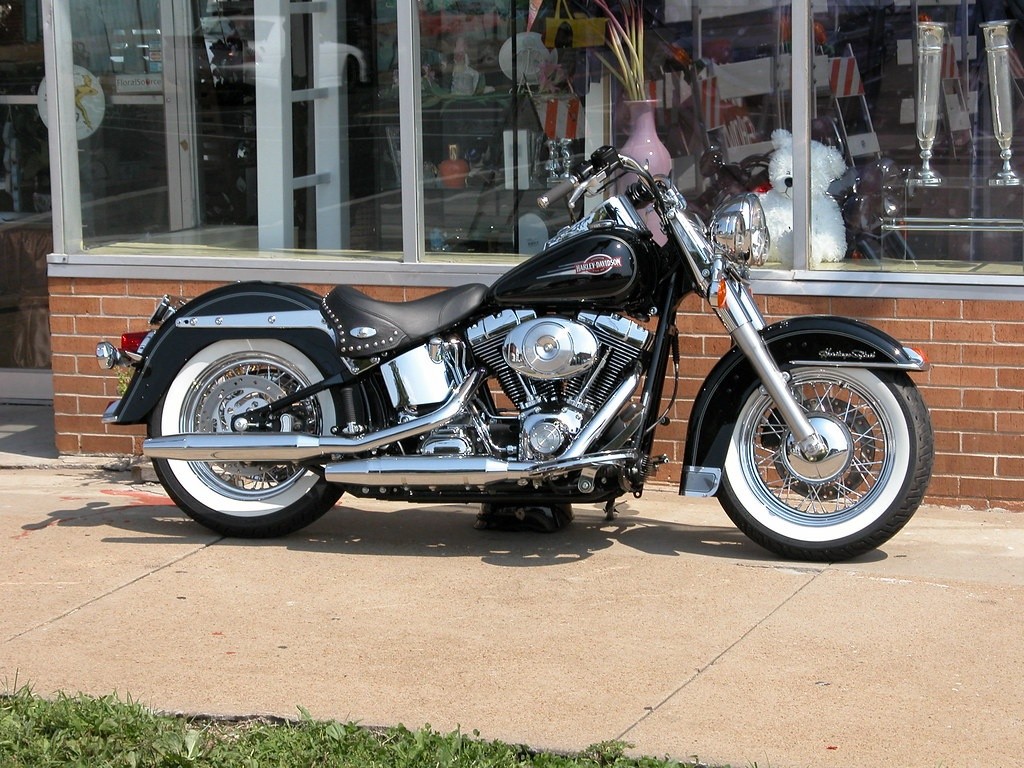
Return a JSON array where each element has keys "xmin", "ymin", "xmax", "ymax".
[{"xmin": 720, "ymin": 128, "xmax": 849, "ymax": 268}]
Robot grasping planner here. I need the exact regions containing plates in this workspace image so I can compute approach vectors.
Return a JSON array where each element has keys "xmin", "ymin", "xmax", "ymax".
[{"xmin": 499, "ymin": 32, "xmax": 558, "ymax": 85}]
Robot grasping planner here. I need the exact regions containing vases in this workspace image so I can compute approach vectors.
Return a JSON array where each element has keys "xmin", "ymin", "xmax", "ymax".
[{"xmin": 616, "ymin": 99, "xmax": 673, "ymax": 247}]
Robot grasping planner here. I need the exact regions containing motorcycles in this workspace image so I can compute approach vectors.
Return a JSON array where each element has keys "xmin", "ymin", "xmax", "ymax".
[
  {"xmin": 695, "ymin": 119, "xmax": 915, "ymax": 262},
  {"xmin": 97, "ymin": 145, "xmax": 934, "ymax": 564}
]
